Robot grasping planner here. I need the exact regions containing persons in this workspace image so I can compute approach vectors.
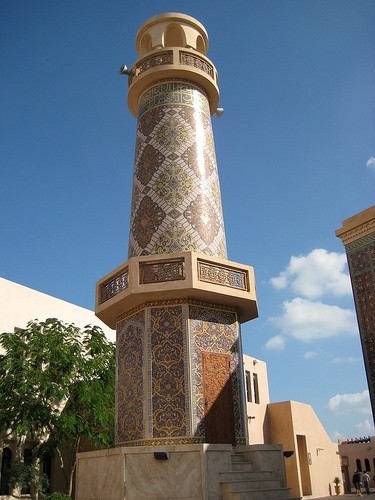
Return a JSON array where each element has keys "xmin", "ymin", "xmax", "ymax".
[{"xmin": 350, "ymin": 469, "xmax": 371, "ymax": 496}]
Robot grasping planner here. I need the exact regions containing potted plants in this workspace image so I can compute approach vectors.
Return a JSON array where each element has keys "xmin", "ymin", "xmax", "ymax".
[{"xmin": 333, "ymin": 477, "xmax": 342, "ymax": 494}]
